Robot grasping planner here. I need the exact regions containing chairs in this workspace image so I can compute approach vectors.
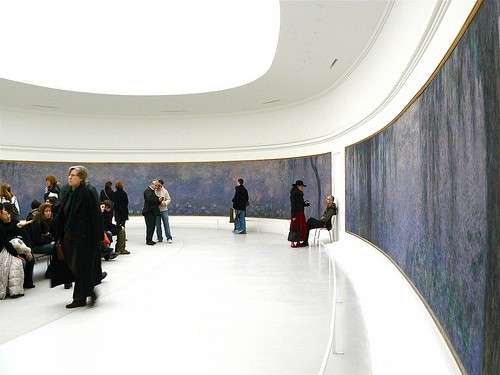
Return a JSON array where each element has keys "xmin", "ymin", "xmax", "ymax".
[{"xmin": 314, "ymin": 215, "xmax": 336, "ymax": 243}]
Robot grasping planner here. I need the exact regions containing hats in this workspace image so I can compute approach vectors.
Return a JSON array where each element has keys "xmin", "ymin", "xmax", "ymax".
[{"xmin": 292, "ymin": 180, "xmax": 307, "ymax": 187}]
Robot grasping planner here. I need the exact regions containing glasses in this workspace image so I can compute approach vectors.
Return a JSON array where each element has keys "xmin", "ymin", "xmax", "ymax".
[{"xmin": 68, "ymin": 174, "xmax": 79, "ymax": 176}]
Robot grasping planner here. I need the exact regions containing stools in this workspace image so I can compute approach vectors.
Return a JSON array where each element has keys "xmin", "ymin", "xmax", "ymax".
[{"xmin": 32, "ymin": 254, "xmax": 53, "ymax": 265}]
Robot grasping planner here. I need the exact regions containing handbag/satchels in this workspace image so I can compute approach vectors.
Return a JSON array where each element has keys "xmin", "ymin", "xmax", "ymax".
[
  {"xmin": 230, "ymin": 209, "xmax": 236, "ymax": 222},
  {"xmin": 56, "ymin": 243, "xmax": 65, "ymax": 262},
  {"xmin": 288, "ymin": 221, "xmax": 300, "ymax": 242}
]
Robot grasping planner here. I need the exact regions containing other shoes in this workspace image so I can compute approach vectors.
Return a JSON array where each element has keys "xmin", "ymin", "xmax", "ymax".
[
  {"xmin": 102, "ymin": 248, "xmax": 113, "ymax": 256},
  {"xmin": 296, "ymin": 243, "xmax": 302, "ymax": 246},
  {"xmin": 66, "ymin": 300, "xmax": 86, "ymax": 308},
  {"xmin": 291, "ymin": 244, "xmax": 297, "ymax": 248},
  {"xmin": 152, "ymin": 241, "xmax": 156, "ymax": 244},
  {"xmin": 45, "ymin": 274, "xmax": 51, "ymax": 278},
  {"xmin": 65, "ymin": 283, "xmax": 72, "ymax": 289},
  {"xmin": 168, "ymin": 239, "xmax": 173, "ymax": 243},
  {"xmin": 101, "ymin": 272, "xmax": 107, "ymax": 280},
  {"xmin": 147, "ymin": 242, "xmax": 154, "ymax": 245},
  {"xmin": 23, "ymin": 284, "xmax": 35, "ymax": 288},
  {"xmin": 158, "ymin": 239, "xmax": 162, "ymax": 242},
  {"xmin": 122, "ymin": 250, "xmax": 130, "ymax": 254},
  {"xmin": 301, "ymin": 241, "xmax": 308, "ymax": 247},
  {"xmin": 104, "ymin": 253, "xmax": 118, "ymax": 261}
]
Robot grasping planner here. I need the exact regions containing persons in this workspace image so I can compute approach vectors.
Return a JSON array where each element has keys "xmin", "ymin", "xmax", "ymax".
[
  {"xmin": 142, "ymin": 180, "xmax": 162, "ymax": 245},
  {"xmin": 232, "ymin": 179, "xmax": 249, "ymax": 234},
  {"xmin": 155, "ymin": 180, "xmax": 172, "ymax": 243},
  {"xmin": 290, "ymin": 180, "xmax": 307, "ymax": 248},
  {"xmin": 301, "ymin": 195, "xmax": 336, "ymax": 247},
  {"xmin": 0, "ymin": 165, "xmax": 130, "ymax": 308}
]
